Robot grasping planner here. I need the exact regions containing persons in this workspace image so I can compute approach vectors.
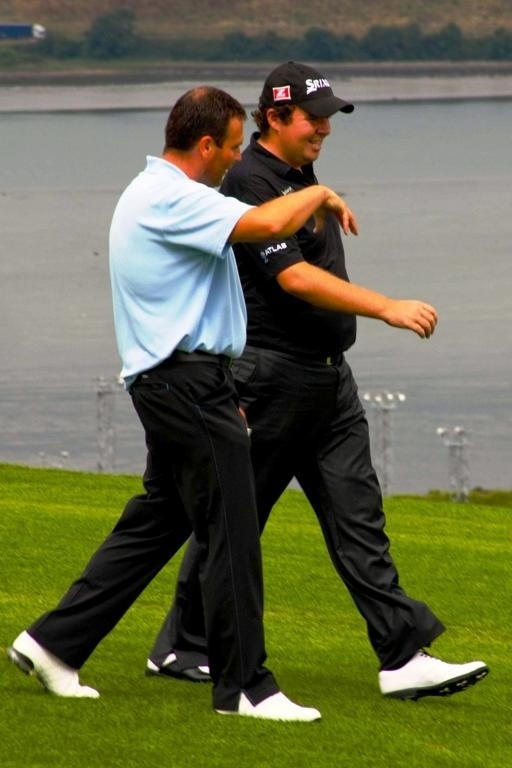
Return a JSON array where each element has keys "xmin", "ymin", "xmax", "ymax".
[
  {"xmin": 140, "ymin": 58, "xmax": 491, "ymax": 705},
  {"xmin": 5, "ymin": 81, "xmax": 360, "ymax": 725}
]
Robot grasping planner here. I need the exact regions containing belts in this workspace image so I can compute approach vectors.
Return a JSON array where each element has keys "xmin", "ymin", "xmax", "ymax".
[{"xmin": 167, "ymin": 349, "xmax": 220, "ymax": 363}]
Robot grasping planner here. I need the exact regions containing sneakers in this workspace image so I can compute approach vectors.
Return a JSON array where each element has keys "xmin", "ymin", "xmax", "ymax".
[
  {"xmin": 377, "ymin": 649, "xmax": 489, "ymax": 701},
  {"xmin": 214, "ymin": 692, "xmax": 321, "ymax": 722},
  {"xmin": 259, "ymin": 60, "xmax": 354, "ymax": 118},
  {"xmin": 5, "ymin": 630, "xmax": 100, "ymax": 698},
  {"xmin": 146, "ymin": 652, "xmax": 211, "ymax": 682}
]
